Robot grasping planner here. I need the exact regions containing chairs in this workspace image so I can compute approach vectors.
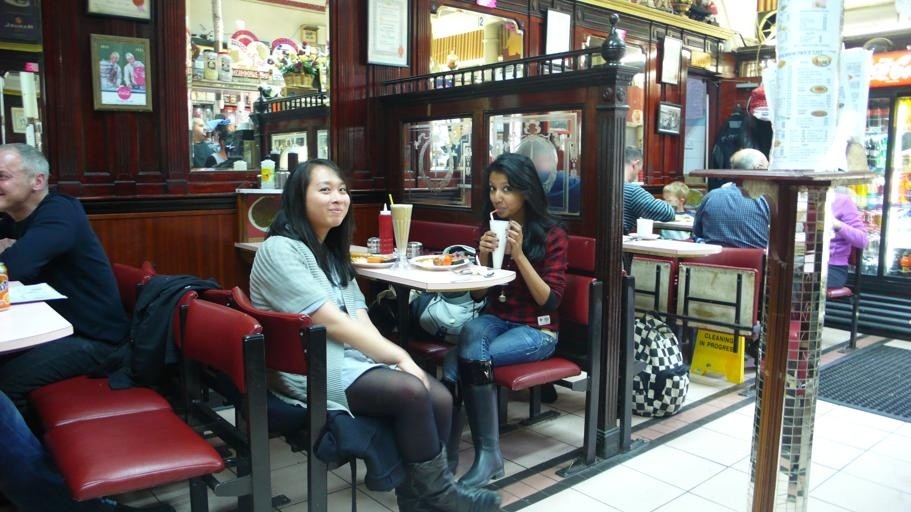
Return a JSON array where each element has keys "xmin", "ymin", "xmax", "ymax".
[
  {"xmin": 827, "ymin": 245, "xmax": 865, "ymax": 347},
  {"xmin": 30, "ymin": 269, "xmax": 200, "ymax": 429},
  {"xmin": 627, "ymin": 235, "xmax": 697, "ymax": 334},
  {"xmin": 670, "ymin": 241, "xmax": 766, "ymax": 373},
  {"xmin": 232, "ymin": 286, "xmax": 393, "ymax": 511},
  {"xmin": 492, "ymin": 233, "xmax": 606, "ymax": 466},
  {"xmin": 39, "ymin": 298, "xmax": 274, "ymax": 511},
  {"xmin": 388, "ymin": 219, "xmax": 482, "ymax": 379},
  {"xmin": 112, "ymin": 264, "xmax": 139, "ymax": 304}
]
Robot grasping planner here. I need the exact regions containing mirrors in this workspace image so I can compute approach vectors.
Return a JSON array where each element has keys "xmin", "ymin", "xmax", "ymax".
[
  {"xmin": 415, "ymin": 2, "xmax": 530, "ymax": 176},
  {"xmin": 1, "ymin": 71, "xmax": 46, "ymax": 154},
  {"xmin": 184, "ymin": 0, "xmax": 331, "ymax": 172},
  {"xmin": 585, "ymin": 34, "xmax": 644, "ymax": 184}
]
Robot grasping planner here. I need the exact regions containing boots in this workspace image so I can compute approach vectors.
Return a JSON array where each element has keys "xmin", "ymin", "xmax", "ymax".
[
  {"xmin": 396, "ymin": 487, "xmax": 439, "ymax": 512},
  {"xmin": 457, "ymin": 383, "xmax": 505, "ymax": 490},
  {"xmin": 402, "ymin": 443, "xmax": 503, "ymax": 512}
]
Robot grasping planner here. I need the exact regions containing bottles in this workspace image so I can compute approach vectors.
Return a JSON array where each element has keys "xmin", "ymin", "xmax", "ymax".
[
  {"xmin": 218, "ymin": 52, "xmax": 233, "ymax": 81},
  {"xmin": 378, "ymin": 203, "xmax": 392, "ymax": 253},
  {"xmin": 0, "ymin": 264, "xmax": 8, "ymax": 310},
  {"xmin": 261, "ymin": 155, "xmax": 275, "ymax": 188},
  {"xmin": 203, "ymin": 49, "xmax": 219, "ymax": 80}
]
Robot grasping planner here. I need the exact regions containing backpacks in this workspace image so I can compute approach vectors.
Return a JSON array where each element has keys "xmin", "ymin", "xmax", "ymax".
[{"xmin": 632, "ymin": 310, "xmax": 688, "ymax": 419}]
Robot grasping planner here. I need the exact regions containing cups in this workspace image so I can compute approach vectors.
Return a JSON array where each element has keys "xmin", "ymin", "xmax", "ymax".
[
  {"xmin": 487, "ymin": 220, "xmax": 511, "ymax": 272},
  {"xmin": 391, "ymin": 204, "xmax": 414, "ymax": 274}
]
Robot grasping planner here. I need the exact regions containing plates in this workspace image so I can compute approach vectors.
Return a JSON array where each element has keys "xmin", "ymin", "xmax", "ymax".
[
  {"xmin": 350, "ymin": 253, "xmax": 396, "ymax": 269},
  {"xmin": 628, "ymin": 233, "xmax": 662, "ymax": 240},
  {"xmin": 408, "ymin": 254, "xmax": 471, "ymax": 271}
]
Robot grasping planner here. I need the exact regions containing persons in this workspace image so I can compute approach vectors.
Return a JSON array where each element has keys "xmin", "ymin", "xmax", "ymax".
[
  {"xmin": 106, "ymin": 52, "xmax": 122, "ymax": 88},
  {"xmin": 688, "ymin": 149, "xmax": 770, "ymax": 250},
  {"xmin": 1, "ymin": 393, "xmax": 171, "ymax": 511},
  {"xmin": 824, "ymin": 180, "xmax": 867, "ymax": 290},
  {"xmin": 442, "ymin": 153, "xmax": 568, "ymax": 488},
  {"xmin": 516, "ymin": 137, "xmax": 579, "ymax": 215},
  {"xmin": 205, "ymin": 130, "xmax": 228, "ymax": 168},
  {"xmin": 658, "ymin": 181, "xmax": 694, "ymax": 240},
  {"xmin": 623, "ymin": 144, "xmax": 674, "ymax": 235},
  {"xmin": 192, "ymin": 117, "xmax": 216, "ymax": 168},
  {"xmin": 249, "ymin": 160, "xmax": 501, "ymax": 511},
  {"xmin": 1, "ymin": 142, "xmax": 126, "ymax": 414},
  {"xmin": 123, "ymin": 52, "xmax": 145, "ymax": 90}
]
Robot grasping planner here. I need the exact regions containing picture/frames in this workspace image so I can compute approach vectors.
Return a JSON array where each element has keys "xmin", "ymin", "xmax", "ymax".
[
  {"xmin": 656, "ymin": 101, "xmax": 684, "ymax": 137},
  {"xmin": 91, "ymin": 33, "xmax": 155, "ymax": 111},
  {"xmin": 658, "ymin": 35, "xmax": 684, "ymax": 87},
  {"xmin": 541, "ymin": 6, "xmax": 575, "ymax": 73},
  {"xmin": 362, "ymin": 1, "xmax": 414, "ymax": 69}
]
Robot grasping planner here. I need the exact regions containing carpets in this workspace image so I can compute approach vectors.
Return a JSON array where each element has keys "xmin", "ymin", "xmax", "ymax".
[{"xmin": 817, "ymin": 343, "xmax": 911, "ymax": 424}]
